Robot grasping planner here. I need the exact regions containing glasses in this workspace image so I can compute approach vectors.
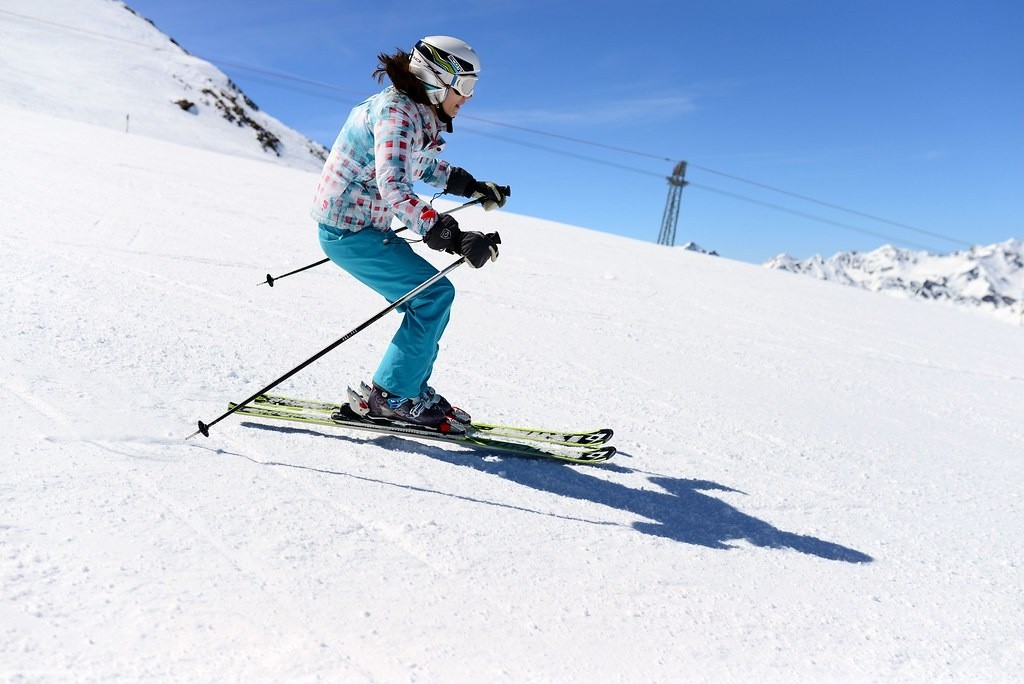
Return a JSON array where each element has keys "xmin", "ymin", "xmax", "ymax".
[{"xmin": 450, "ymin": 74, "xmax": 479, "ymax": 97}]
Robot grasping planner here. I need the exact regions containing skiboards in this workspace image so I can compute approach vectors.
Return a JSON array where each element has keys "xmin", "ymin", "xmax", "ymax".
[{"xmin": 226, "ymin": 391, "xmax": 617, "ymax": 464}]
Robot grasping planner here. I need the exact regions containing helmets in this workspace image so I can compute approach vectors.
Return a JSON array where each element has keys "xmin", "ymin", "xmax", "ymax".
[{"xmin": 408, "ymin": 35, "xmax": 481, "ymax": 104}]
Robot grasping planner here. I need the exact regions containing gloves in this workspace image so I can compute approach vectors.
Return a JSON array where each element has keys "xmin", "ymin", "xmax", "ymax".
[
  {"xmin": 443, "ymin": 166, "xmax": 507, "ymax": 212},
  {"xmin": 423, "ymin": 213, "xmax": 499, "ymax": 269}
]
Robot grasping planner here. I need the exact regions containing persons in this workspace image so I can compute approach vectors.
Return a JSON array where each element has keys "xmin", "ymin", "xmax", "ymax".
[{"xmin": 307, "ymin": 35, "xmax": 503, "ymax": 436}]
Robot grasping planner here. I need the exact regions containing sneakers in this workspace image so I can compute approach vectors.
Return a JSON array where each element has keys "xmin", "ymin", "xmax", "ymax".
[{"xmin": 366, "ymin": 381, "xmax": 455, "ymax": 431}]
